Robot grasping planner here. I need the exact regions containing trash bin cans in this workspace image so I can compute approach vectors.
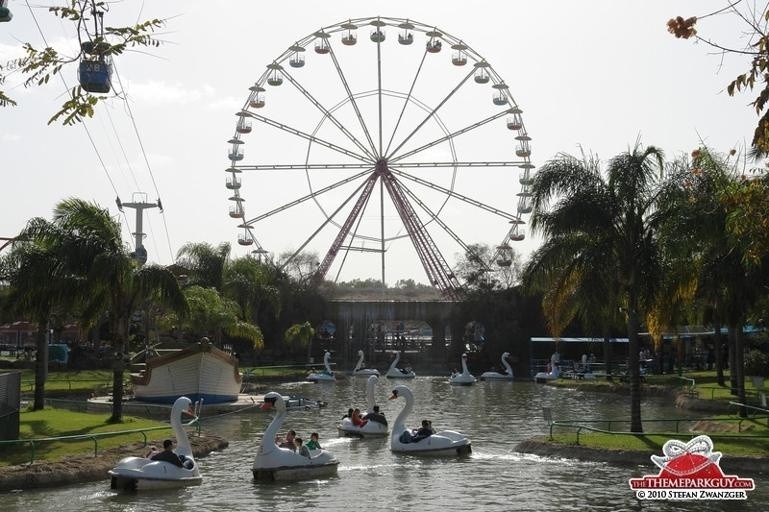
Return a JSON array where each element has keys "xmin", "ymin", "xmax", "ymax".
[{"xmin": 47, "ymin": 344, "xmax": 68, "ymax": 373}]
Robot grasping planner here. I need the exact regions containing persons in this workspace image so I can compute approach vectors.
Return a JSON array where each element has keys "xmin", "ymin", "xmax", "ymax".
[
  {"xmin": 342, "ymin": 406, "xmax": 387, "ymax": 427},
  {"xmin": 276, "ymin": 430, "xmax": 296, "ymax": 453},
  {"xmin": 411, "ymin": 420, "xmax": 432, "ymax": 442},
  {"xmin": 295, "ymin": 438, "xmax": 310, "ymax": 460},
  {"xmin": 428, "ymin": 421, "xmax": 436, "ymax": 434},
  {"xmin": 305, "ymin": 433, "xmax": 321, "ymax": 450},
  {"xmin": 151, "ymin": 440, "xmax": 193, "ymax": 469}
]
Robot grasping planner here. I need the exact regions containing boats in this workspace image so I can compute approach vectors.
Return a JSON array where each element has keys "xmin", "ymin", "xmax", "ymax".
[
  {"xmin": 306, "ymin": 350, "xmax": 336, "ymax": 381},
  {"xmin": 337, "ymin": 375, "xmax": 390, "ymax": 440},
  {"xmin": 250, "ymin": 391, "xmax": 340, "ymax": 482},
  {"xmin": 387, "ymin": 384, "xmax": 474, "ymax": 458},
  {"xmin": 387, "ymin": 350, "xmax": 416, "ymax": 378},
  {"xmin": 109, "ymin": 395, "xmax": 203, "ymax": 493},
  {"xmin": 130, "ymin": 337, "xmax": 243, "ymax": 404},
  {"xmin": 479, "ymin": 352, "xmax": 515, "ymax": 380},
  {"xmin": 533, "ymin": 352, "xmax": 597, "ymax": 383},
  {"xmin": 447, "ymin": 351, "xmax": 478, "ymax": 385},
  {"xmin": 350, "ymin": 349, "xmax": 381, "ymax": 376}
]
